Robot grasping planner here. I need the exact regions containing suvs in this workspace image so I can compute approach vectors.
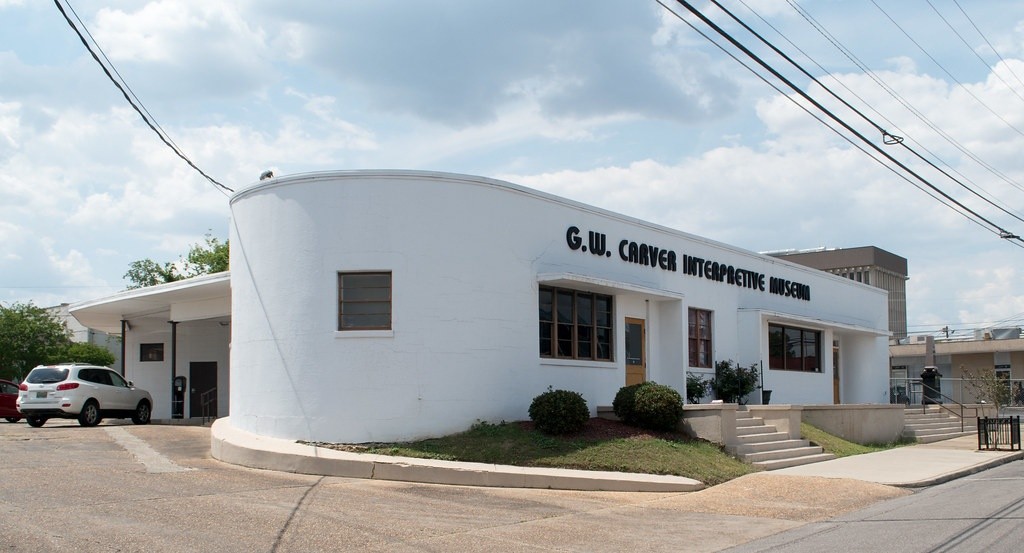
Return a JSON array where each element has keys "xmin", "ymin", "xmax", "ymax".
[{"xmin": 16, "ymin": 365, "xmax": 153, "ymax": 427}]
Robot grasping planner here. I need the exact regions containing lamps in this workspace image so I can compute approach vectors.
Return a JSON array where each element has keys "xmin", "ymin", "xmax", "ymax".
[{"xmin": 220, "ymin": 321, "xmax": 230, "ymax": 326}]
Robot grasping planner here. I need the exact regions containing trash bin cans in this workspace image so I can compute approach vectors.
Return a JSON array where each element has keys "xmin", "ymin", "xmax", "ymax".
[
  {"xmin": 762, "ymin": 390, "xmax": 772, "ymax": 405},
  {"xmin": 921, "ymin": 366, "xmax": 943, "ymax": 404}
]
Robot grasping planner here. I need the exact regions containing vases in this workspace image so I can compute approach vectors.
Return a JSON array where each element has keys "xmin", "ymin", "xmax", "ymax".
[{"xmin": 763, "ymin": 390, "xmax": 772, "ymax": 404}]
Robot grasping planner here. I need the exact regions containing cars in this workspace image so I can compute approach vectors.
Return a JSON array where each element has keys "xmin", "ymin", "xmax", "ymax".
[
  {"xmin": 890, "ymin": 386, "xmax": 911, "ymax": 407},
  {"xmin": 0, "ymin": 379, "xmax": 27, "ymax": 423}
]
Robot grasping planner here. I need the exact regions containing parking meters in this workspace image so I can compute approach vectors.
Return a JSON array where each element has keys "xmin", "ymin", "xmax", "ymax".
[{"xmin": 174, "ymin": 376, "xmax": 186, "ymax": 418}]
